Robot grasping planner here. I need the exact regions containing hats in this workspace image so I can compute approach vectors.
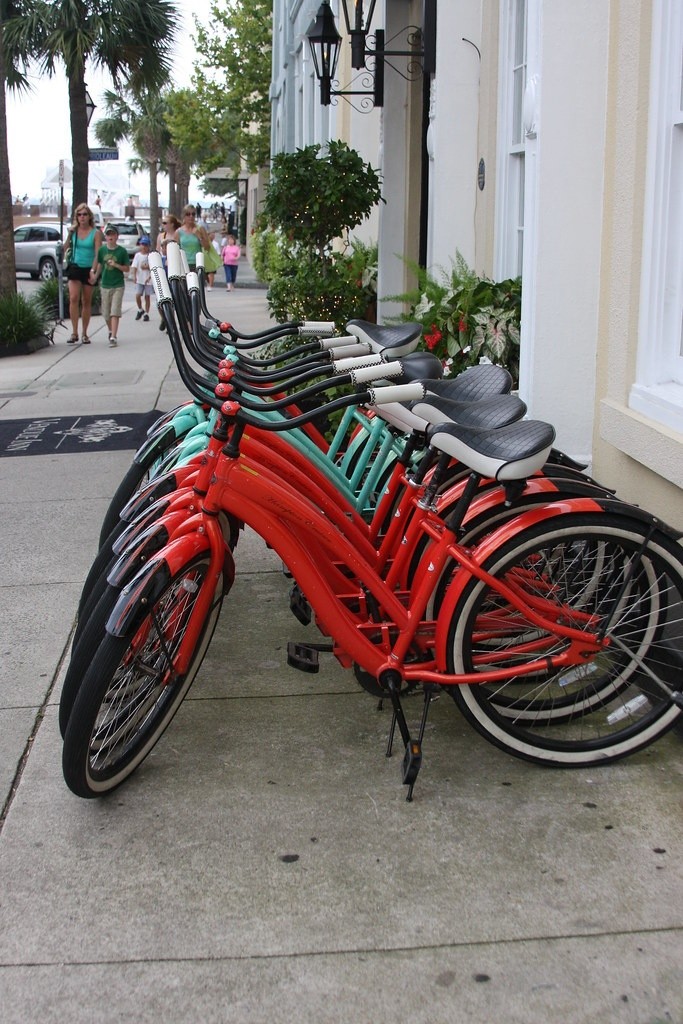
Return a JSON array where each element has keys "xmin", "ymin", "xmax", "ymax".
[
  {"xmin": 104, "ymin": 225, "xmax": 118, "ymax": 235},
  {"xmin": 139, "ymin": 237, "xmax": 150, "ymax": 244}
]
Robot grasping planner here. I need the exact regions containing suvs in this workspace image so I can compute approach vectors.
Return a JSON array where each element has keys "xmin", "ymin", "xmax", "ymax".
[
  {"xmin": 103, "ymin": 221, "xmax": 149, "ymax": 255},
  {"xmin": 14, "ymin": 221, "xmax": 71, "ymax": 281}
]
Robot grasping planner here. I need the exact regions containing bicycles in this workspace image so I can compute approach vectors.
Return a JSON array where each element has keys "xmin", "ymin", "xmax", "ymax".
[{"xmin": 56, "ymin": 242, "xmax": 683, "ymax": 806}]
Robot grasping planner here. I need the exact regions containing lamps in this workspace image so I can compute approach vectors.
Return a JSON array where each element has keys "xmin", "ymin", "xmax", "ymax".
[{"xmin": 305, "ymin": 0, "xmax": 384, "ymax": 115}]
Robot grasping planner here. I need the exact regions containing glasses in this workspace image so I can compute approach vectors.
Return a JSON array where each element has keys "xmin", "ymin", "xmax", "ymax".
[
  {"xmin": 78, "ymin": 213, "xmax": 88, "ymax": 216},
  {"xmin": 187, "ymin": 212, "xmax": 195, "ymax": 216},
  {"xmin": 162, "ymin": 222, "xmax": 170, "ymax": 225}
]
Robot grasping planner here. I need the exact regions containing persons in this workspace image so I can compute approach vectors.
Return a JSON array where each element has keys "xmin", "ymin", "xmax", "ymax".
[
  {"xmin": 16, "ymin": 194, "xmax": 30, "ymax": 209},
  {"xmin": 64, "ymin": 204, "xmax": 102, "ymax": 343},
  {"xmin": 96, "ymin": 196, "xmax": 101, "ymax": 210},
  {"xmin": 132, "ymin": 237, "xmax": 154, "ymax": 321},
  {"xmin": 221, "ymin": 233, "xmax": 240, "ymax": 291},
  {"xmin": 88, "ymin": 226, "xmax": 130, "ymax": 347},
  {"xmin": 128, "ymin": 197, "xmax": 133, "ymax": 206},
  {"xmin": 156, "ymin": 205, "xmax": 210, "ymax": 333},
  {"xmin": 195, "ymin": 202, "xmax": 228, "ymax": 291}
]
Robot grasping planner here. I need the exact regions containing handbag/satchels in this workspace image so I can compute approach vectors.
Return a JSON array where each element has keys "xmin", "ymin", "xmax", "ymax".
[
  {"xmin": 197, "ymin": 224, "xmax": 221, "ymax": 273},
  {"xmin": 65, "ymin": 263, "xmax": 78, "ymax": 279}
]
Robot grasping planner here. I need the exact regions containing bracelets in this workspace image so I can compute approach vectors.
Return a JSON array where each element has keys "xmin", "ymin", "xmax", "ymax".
[
  {"xmin": 91, "ymin": 269, "xmax": 96, "ymax": 272},
  {"xmin": 199, "ymin": 237, "xmax": 203, "ymax": 240}
]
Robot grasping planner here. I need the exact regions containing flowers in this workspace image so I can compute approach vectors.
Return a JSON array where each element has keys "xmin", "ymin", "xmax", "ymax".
[{"xmin": 379, "ymin": 247, "xmax": 521, "ymax": 390}]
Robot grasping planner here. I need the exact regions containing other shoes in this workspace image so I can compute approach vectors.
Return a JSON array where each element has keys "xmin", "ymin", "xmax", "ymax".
[
  {"xmin": 108, "ymin": 337, "xmax": 117, "ymax": 346},
  {"xmin": 135, "ymin": 310, "xmax": 144, "ymax": 320},
  {"xmin": 144, "ymin": 315, "xmax": 149, "ymax": 321},
  {"xmin": 226, "ymin": 287, "xmax": 234, "ymax": 292},
  {"xmin": 207, "ymin": 286, "xmax": 213, "ymax": 292},
  {"xmin": 82, "ymin": 335, "xmax": 91, "ymax": 343},
  {"xmin": 67, "ymin": 334, "xmax": 78, "ymax": 343}
]
